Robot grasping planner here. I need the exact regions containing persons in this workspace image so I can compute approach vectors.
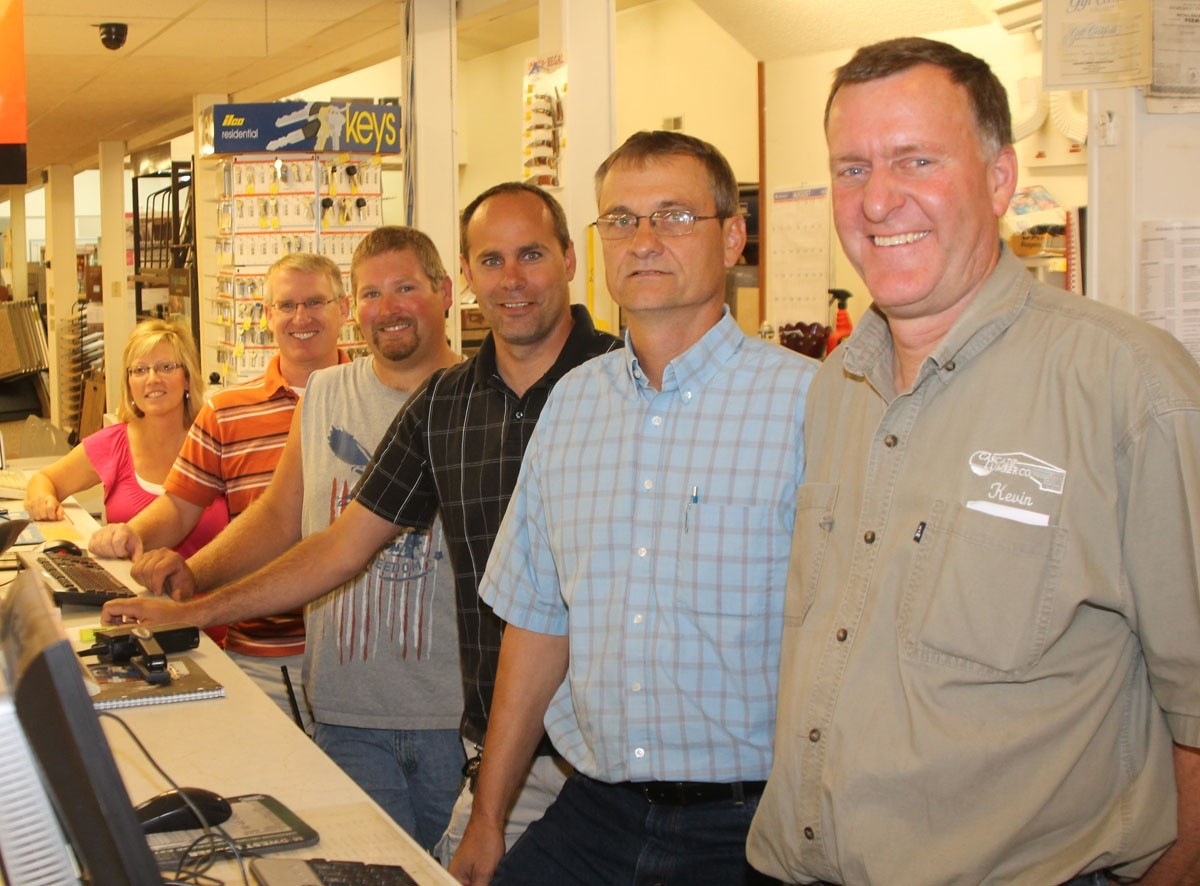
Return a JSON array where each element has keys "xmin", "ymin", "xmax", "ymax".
[
  {"xmin": 131, "ymin": 227, "xmax": 470, "ymax": 864},
  {"xmin": 90, "ymin": 252, "xmax": 353, "ymax": 742},
  {"xmin": 23, "ymin": 318, "xmax": 229, "ymax": 653},
  {"xmin": 100, "ymin": 183, "xmax": 627, "ymax": 860},
  {"xmin": 746, "ymin": 37, "xmax": 1200, "ymax": 886},
  {"xmin": 445, "ymin": 131, "xmax": 824, "ymax": 886}
]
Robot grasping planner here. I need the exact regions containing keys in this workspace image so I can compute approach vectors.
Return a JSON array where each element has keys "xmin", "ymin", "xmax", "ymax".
[{"xmin": 216, "ymin": 157, "xmax": 382, "ymax": 368}]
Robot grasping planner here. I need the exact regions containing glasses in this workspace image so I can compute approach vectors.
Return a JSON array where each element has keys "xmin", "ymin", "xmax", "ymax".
[
  {"xmin": 588, "ymin": 208, "xmax": 728, "ymax": 240},
  {"xmin": 129, "ymin": 360, "xmax": 184, "ymax": 375},
  {"xmin": 265, "ymin": 296, "xmax": 339, "ymax": 312}
]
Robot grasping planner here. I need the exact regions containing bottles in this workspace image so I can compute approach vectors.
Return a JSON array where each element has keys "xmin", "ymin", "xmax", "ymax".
[{"xmin": 757, "ymin": 321, "xmax": 775, "ymax": 341}]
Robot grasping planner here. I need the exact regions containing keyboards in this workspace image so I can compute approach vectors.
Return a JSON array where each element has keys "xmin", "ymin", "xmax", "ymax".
[
  {"xmin": 250, "ymin": 858, "xmax": 419, "ymax": 886},
  {"xmin": 15, "ymin": 552, "xmax": 137, "ymax": 608},
  {"xmin": 0, "ymin": 469, "xmax": 28, "ymax": 499}
]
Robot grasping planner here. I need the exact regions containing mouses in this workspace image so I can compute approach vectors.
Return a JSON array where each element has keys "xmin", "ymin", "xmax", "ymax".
[
  {"xmin": 33, "ymin": 540, "xmax": 82, "ymax": 553},
  {"xmin": 134, "ymin": 787, "xmax": 232, "ymax": 834}
]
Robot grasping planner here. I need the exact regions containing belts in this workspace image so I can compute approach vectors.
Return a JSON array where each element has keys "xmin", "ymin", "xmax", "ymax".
[{"xmin": 224, "ymin": 640, "xmax": 307, "ymax": 656}]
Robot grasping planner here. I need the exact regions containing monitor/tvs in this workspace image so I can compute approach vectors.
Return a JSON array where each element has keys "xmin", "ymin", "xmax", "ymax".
[{"xmin": 0, "ymin": 566, "xmax": 165, "ymax": 886}]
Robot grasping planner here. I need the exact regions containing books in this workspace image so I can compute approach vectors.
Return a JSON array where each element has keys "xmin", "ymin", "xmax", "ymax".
[{"xmin": 84, "ymin": 652, "xmax": 226, "ymax": 710}]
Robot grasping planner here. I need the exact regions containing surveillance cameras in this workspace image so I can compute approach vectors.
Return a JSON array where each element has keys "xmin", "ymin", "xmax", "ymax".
[{"xmin": 98, "ymin": 23, "xmax": 127, "ymax": 50}]
